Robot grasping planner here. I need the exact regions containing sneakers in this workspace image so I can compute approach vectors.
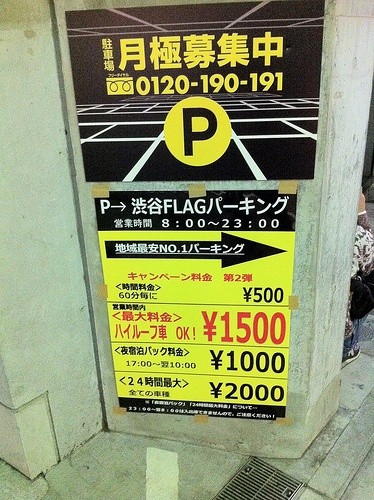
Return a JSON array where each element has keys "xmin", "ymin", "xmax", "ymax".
[{"xmin": 340, "ymin": 342, "xmax": 360, "ymax": 369}]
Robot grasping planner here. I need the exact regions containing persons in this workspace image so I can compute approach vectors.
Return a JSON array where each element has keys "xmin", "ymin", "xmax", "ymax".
[{"xmin": 342, "ymin": 183, "xmax": 374, "ymax": 368}]
